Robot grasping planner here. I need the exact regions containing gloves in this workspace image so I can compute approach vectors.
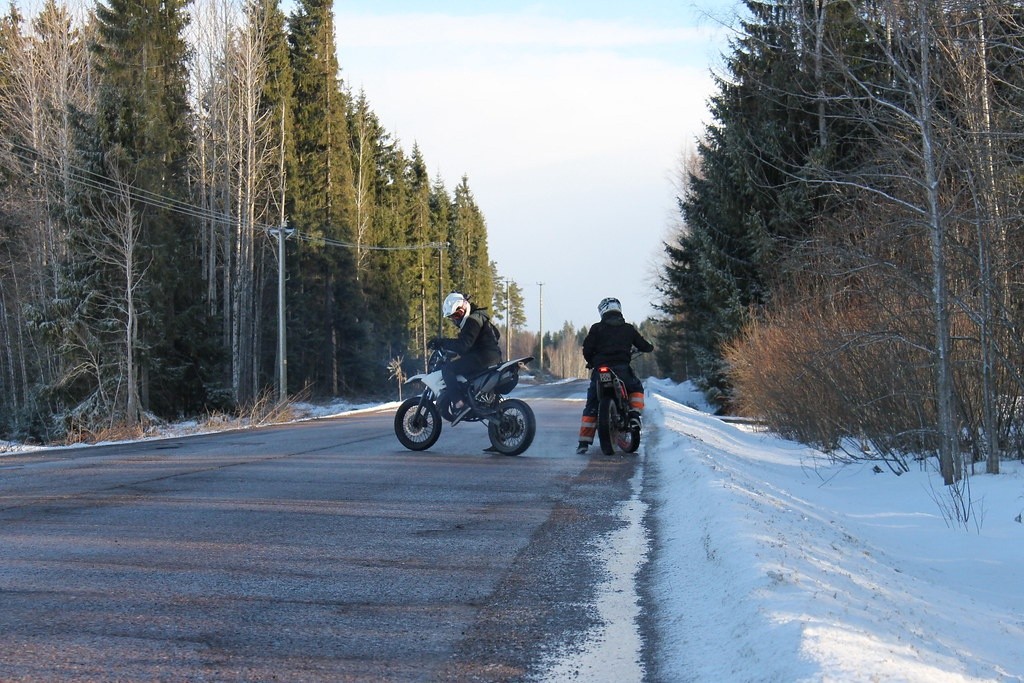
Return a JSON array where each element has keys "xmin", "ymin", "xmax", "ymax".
[{"xmin": 427, "ymin": 338, "xmax": 443, "ymax": 350}]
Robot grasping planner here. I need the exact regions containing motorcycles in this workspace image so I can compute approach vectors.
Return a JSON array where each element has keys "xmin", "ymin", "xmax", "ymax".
[
  {"xmin": 394, "ymin": 342, "xmax": 537, "ymax": 457},
  {"xmin": 585, "ymin": 347, "xmax": 652, "ymax": 456}
]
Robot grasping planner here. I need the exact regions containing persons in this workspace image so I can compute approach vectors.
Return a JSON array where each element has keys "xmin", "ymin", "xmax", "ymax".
[
  {"xmin": 427, "ymin": 291, "xmax": 502, "ymax": 427},
  {"xmin": 576, "ymin": 298, "xmax": 654, "ymax": 454}
]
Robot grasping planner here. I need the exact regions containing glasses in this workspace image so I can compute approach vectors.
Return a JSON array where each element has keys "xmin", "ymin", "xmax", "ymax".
[{"xmin": 452, "ymin": 309, "xmax": 464, "ymax": 321}]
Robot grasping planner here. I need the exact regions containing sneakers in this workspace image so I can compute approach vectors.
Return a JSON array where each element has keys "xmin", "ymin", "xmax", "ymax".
[
  {"xmin": 450, "ymin": 399, "xmax": 471, "ymax": 427},
  {"xmin": 630, "ymin": 414, "xmax": 642, "ymax": 431},
  {"xmin": 576, "ymin": 444, "xmax": 588, "ymax": 454}
]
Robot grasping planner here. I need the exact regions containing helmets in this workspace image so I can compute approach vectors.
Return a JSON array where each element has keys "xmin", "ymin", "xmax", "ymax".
[
  {"xmin": 597, "ymin": 297, "xmax": 622, "ymax": 316},
  {"xmin": 443, "ymin": 293, "xmax": 471, "ymax": 330}
]
